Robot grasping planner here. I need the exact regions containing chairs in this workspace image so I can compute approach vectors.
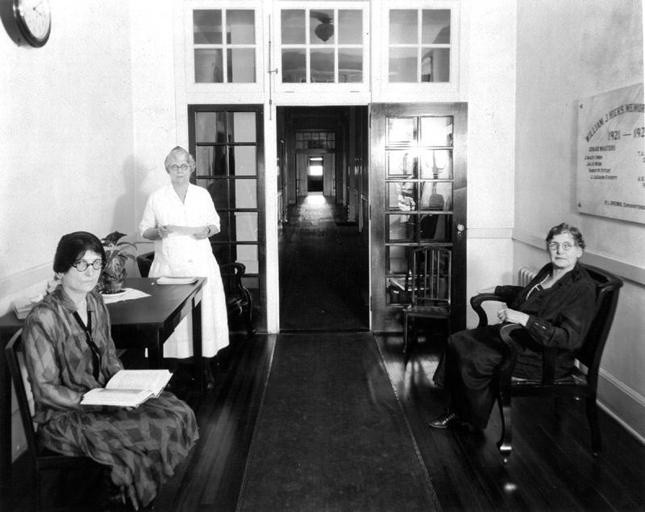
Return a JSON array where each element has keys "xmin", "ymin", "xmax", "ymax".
[
  {"xmin": 470, "ymin": 263, "xmax": 624, "ymax": 464},
  {"xmin": 136, "ymin": 251, "xmax": 258, "ymax": 393},
  {"xmin": 4, "ymin": 328, "xmax": 172, "ymax": 512},
  {"xmin": 400, "ymin": 245, "xmax": 452, "ymax": 354}
]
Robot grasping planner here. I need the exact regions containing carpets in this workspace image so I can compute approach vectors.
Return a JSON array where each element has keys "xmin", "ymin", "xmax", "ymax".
[{"xmin": 234, "ymin": 331, "xmax": 445, "ymax": 512}]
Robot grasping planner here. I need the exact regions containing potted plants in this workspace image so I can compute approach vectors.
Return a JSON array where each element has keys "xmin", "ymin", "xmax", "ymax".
[{"xmin": 95, "ymin": 230, "xmax": 154, "ymax": 297}]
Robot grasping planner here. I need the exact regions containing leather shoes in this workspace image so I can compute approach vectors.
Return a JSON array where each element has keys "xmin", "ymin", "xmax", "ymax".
[
  {"xmin": 201, "ymin": 357, "xmax": 214, "ymax": 390},
  {"xmin": 427, "ymin": 407, "xmax": 459, "ymax": 429}
]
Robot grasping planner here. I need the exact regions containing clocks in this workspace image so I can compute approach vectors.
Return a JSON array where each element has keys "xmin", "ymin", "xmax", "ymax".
[{"xmin": 0, "ymin": 0, "xmax": 52, "ymax": 48}]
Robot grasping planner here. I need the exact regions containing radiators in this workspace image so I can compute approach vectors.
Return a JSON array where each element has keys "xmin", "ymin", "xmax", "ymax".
[{"xmin": 518, "ymin": 266, "xmax": 536, "ymax": 288}]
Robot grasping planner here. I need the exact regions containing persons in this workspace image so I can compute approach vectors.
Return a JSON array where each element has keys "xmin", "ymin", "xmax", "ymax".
[
  {"xmin": 22, "ymin": 230, "xmax": 201, "ymax": 511},
  {"xmin": 427, "ymin": 223, "xmax": 597, "ymax": 430},
  {"xmin": 138, "ymin": 146, "xmax": 231, "ymax": 360}
]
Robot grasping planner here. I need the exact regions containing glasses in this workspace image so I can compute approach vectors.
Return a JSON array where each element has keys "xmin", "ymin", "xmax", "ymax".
[
  {"xmin": 170, "ymin": 164, "xmax": 192, "ymax": 171},
  {"xmin": 71, "ymin": 259, "xmax": 104, "ymax": 272},
  {"xmin": 549, "ymin": 243, "xmax": 574, "ymax": 250}
]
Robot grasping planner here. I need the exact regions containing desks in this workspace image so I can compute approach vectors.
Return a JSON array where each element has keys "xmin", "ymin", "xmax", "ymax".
[{"xmin": 0, "ymin": 276, "xmax": 207, "ymax": 483}]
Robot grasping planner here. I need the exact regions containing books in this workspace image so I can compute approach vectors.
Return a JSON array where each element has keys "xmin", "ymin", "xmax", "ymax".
[{"xmin": 78, "ymin": 368, "xmax": 175, "ymax": 406}]
